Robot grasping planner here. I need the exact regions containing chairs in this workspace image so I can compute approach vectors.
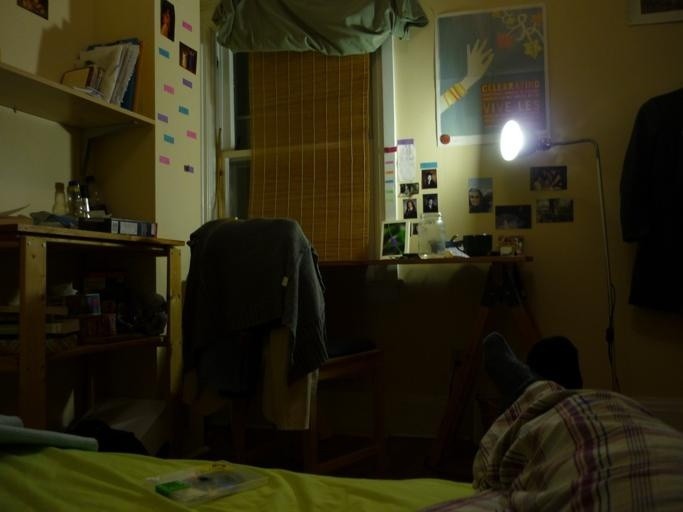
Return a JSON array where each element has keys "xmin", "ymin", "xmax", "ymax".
[{"xmin": 187, "ymin": 219, "xmax": 385, "ymax": 475}]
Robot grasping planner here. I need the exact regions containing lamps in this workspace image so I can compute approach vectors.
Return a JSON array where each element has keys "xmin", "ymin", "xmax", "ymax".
[{"xmin": 499, "ymin": 118, "xmax": 620, "ymax": 391}]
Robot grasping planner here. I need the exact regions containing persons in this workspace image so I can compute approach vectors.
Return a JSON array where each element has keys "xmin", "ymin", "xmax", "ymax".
[
  {"xmin": 469, "ymin": 189, "xmax": 491, "ymax": 212},
  {"xmin": 423, "ymin": 174, "xmax": 437, "ymax": 188},
  {"xmin": 424, "ymin": 198, "xmax": 438, "ymax": 212},
  {"xmin": 439, "ymin": 38, "xmax": 495, "ymax": 114},
  {"xmin": 404, "ymin": 201, "xmax": 417, "ymax": 218},
  {"xmin": 162, "ymin": 13, "xmax": 169, "ymax": 37}
]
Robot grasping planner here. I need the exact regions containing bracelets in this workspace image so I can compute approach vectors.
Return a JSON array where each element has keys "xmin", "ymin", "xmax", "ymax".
[{"xmin": 443, "ymin": 82, "xmax": 467, "ymax": 108}]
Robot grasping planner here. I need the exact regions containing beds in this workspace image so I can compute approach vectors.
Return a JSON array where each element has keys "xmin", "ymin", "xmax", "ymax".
[{"xmin": 0, "ymin": 416, "xmax": 683, "ymax": 512}]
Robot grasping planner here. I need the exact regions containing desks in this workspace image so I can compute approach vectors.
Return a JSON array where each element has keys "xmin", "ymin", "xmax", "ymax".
[{"xmin": 313, "ymin": 257, "xmax": 540, "ymax": 466}]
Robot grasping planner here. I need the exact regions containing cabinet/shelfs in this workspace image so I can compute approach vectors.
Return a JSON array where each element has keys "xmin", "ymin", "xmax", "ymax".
[{"xmin": 0, "ymin": 217, "xmax": 184, "ymax": 430}]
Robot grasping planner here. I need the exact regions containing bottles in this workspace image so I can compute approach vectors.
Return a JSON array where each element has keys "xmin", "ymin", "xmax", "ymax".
[
  {"xmin": 67, "ymin": 181, "xmax": 81, "ymax": 217},
  {"xmin": 53, "ymin": 182, "xmax": 66, "ymax": 216},
  {"xmin": 416, "ymin": 213, "xmax": 447, "ymax": 260}
]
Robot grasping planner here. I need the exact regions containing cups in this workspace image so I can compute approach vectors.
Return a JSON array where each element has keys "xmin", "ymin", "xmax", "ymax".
[{"xmin": 455, "ymin": 234, "xmax": 493, "ymax": 257}]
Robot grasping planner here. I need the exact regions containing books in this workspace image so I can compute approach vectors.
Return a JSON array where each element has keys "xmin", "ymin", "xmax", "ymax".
[
  {"xmin": 142, "ymin": 460, "xmax": 270, "ymax": 508},
  {"xmin": 83, "ymin": 217, "xmax": 156, "ymax": 236},
  {"xmin": 60, "ymin": 38, "xmax": 143, "ymax": 113}
]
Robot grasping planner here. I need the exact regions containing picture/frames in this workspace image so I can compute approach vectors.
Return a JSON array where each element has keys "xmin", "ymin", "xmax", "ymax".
[
  {"xmin": 379, "ymin": 219, "xmax": 410, "ymax": 259},
  {"xmin": 624, "ymin": 0, "xmax": 682, "ymax": 26}
]
